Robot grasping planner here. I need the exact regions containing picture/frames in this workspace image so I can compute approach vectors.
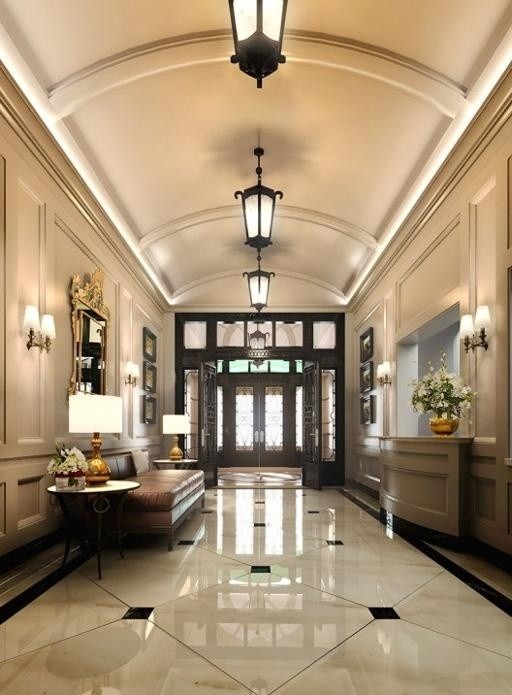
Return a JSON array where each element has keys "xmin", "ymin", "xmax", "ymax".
[
  {"xmin": 360, "ymin": 395, "xmax": 372, "ymax": 425},
  {"xmin": 144, "ymin": 395, "xmax": 157, "ymax": 424},
  {"xmin": 359, "ymin": 361, "xmax": 373, "ymax": 394},
  {"xmin": 143, "ymin": 361, "xmax": 157, "ymax": 393},
  {"xmin": 143, "ymin": 326, "xmax": 157, "ymax": 362},
  {"xmin": 359, "ymin": 327, "xmax": 373, "ymax": 363}
]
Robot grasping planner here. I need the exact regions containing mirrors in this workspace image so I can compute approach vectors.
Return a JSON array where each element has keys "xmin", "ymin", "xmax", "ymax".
[{"xmin": 69, "ymin": 268, "xmax": 112, "ymax": 397}]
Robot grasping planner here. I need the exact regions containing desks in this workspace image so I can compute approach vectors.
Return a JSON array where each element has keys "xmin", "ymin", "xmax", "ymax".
[
  {"xmin": 153, "ymin": 459, "xmax": 198, "ymax": 470},
  {"xmin": 45, "ymin": 480, "xmax": 142, "ymax": 578}
]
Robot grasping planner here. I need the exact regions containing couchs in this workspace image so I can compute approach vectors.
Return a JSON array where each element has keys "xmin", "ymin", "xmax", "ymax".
[{"xmin": 86, "ymin": 450, "xmax": 206, "ymax": 551}]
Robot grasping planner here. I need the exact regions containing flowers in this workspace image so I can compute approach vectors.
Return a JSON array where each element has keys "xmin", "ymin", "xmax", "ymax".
[
  {"xmin": 411, "ymin": 348, "xmax": 476, "ymax": 419},
  {"xmin": 47, "ymin": 441, "xmax": 89, "ymax": 473}
]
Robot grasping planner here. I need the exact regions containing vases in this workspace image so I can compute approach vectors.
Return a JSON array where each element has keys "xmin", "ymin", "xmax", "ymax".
[
  {"xmin": 429, "ymin": 417, "xmax": 460, "ymax": 436},
  {"xmin": 54, "ymin": 473, "xmax": 85, "ymax": 490}
]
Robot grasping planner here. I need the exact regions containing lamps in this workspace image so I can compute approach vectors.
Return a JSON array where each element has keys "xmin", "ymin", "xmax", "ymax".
[
  {"xmin": 234, "ymin": 184, "xmax": 283, "ymax": 252},
  {"xmin": 69, "ymin": 395, "xmax": 123, "ymax": 485},
  {"xmin": 39, "ymin": 313, "xmax": 56, "ymax": 352},
  {"xmin": 161, "ymin": 415, "xmax": 190, "ymax": 460},
  {"xmin": 23, "ymin": 304, "xmax": 41, "ymax": 354},
  {"xmin": 377, "ymin": 361, "xmax": 384, "ymax": 384},
  {"xmin": 226, "ymin": 0, "xmax": 288, "ymax": 90},
  {"xmin": 382, "ymin": 360, "xmax": 390, "ymax": 383},
  {"xmin": 244, "ymin": 264, "xmax": 275, "ymax": 313},
  {"xmin": 127, "ymin": 361, "xmax": 140, "ymax": 385},
  {"xmin": 248, "ymin": 322, "xmax": 270, "ymax": 368},
  {"xmin": 474, "ymin": 303, "xmax": 489, "ymax": 351},
  {"xmin": 461, "ymin": 306, "xmax": 477, "ymax": 353}
]
